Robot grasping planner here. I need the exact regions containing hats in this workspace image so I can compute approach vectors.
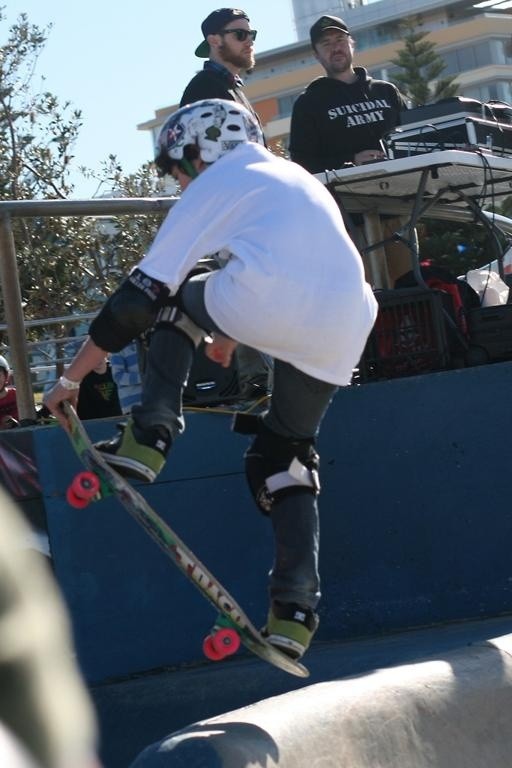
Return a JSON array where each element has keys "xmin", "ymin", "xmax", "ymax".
[
  {"xmin": 311, "ymin": 14, "xmax": 350, "ymax": 42},
  {"xmin": 196, "ymin": 9, "xmax": 249, "ymax": 59}
]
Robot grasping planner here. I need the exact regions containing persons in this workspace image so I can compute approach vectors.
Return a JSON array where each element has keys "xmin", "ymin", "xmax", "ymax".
[
  {"xmin": 0, "ymin": 437, "xmax": 42, "ymax": 499},
  {"xmin": 111, "ymin": 332, "xmax": 149, "ymax": 413},
  {"xmin": 291, "ymin": 16, "xmax": 421, "ymax": 291},
  {"xmin": 36, "ymin": 344, "xmax": 123, "ymax": 420},
  {"xmin": 40, "ymin": 98, "xmax": 380, "ymax": 658},
  {"xmin": 178, "ymin": 8, "xmax": 268, "ymax": 150},
  {"xmin": 0, "ymin": 356, "xmax": 20, "ymax": 430}
]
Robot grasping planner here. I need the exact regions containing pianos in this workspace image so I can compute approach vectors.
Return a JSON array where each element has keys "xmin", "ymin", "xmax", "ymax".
[{"xmin": 400, "ymin": 96, "xmax": 512, "ymax": 132}]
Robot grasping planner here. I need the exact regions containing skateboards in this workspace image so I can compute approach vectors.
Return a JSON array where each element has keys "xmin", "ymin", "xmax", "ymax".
[{"xmin": 56, "ymin": 400, "xmax": 310, "ymax": 678}]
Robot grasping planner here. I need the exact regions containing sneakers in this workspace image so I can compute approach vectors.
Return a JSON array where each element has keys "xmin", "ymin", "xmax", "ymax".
[
  {"xmin": 90, "ymin": 412, "xmax": 172, "ymax": 485},
  {"xmin": 260, "ymin": 598, "xmax": 321, "ymax": 660}
]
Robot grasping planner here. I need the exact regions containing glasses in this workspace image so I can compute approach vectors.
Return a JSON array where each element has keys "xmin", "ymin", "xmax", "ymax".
[{"xmin": 225, "ymin": 29, "xmax": 257, "ymax": 40}]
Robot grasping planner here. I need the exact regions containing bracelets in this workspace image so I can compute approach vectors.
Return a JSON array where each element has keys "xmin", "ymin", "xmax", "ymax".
[{"xmin": 59, "ymin": 374, "xmax": 82, "ymax": 391}]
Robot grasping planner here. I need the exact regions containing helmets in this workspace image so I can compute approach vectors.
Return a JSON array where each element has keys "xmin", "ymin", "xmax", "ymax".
[{"xmin": 155, "ymin": 99, "xmax": 264, "ymax": 184}]
